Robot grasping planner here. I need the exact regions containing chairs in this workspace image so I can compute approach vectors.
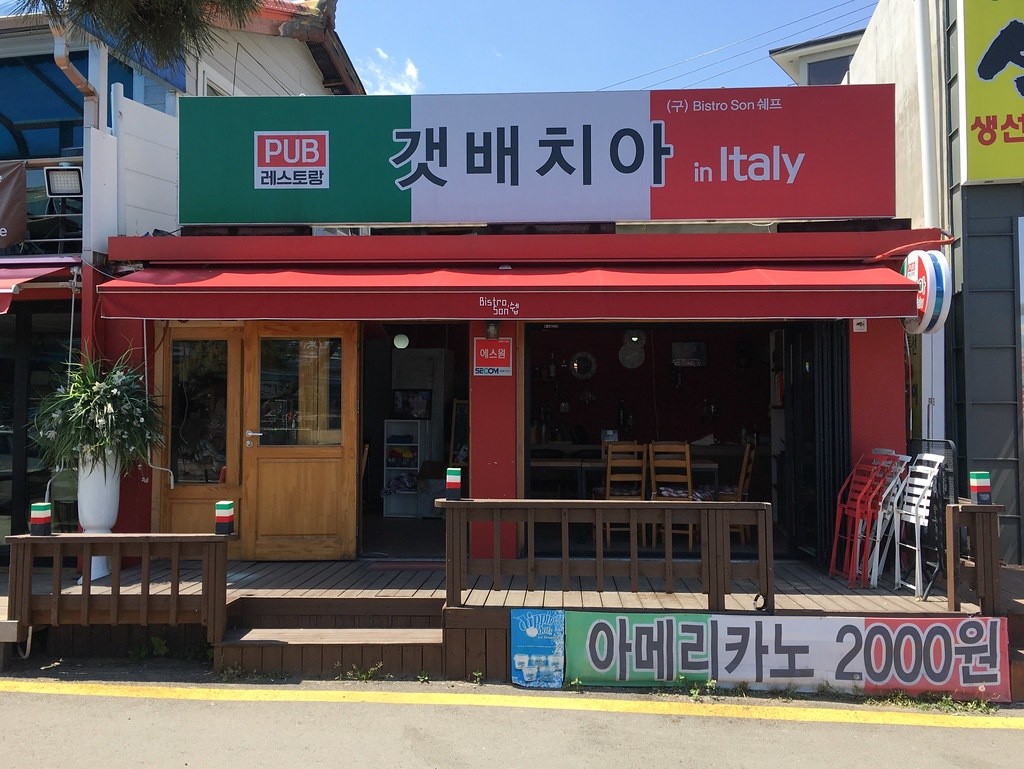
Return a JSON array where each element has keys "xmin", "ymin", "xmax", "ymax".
[
  {"xmin": 650, "ymin": 444, "xmax": 714, "ymax": 550},
  {"xmin": 846, "ymin": 453, "xmax": 900, "ymax": 589},
  {"xmin": 704, "ymin": 443, "xmax": 761, "ymax": 544},
  {"xmin": 593, "ymin": 443, "xmax": 648, "ymax": 547},
  {"xmin": 861, "ymin": 453, "xmax": 911, "ymax": 582},
  {"xmin": 828, "ymin": 455, "xmax": 889, "ymax": 590},
  {"xmin": 853, "ymin": 449, "xmax": 897, "ymax": 588},
  {"xmin": 894, "ymin": 453, "xmax": 946, "ymax": 598}
]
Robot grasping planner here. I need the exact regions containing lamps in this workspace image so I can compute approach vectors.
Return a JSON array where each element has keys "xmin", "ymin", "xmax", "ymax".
[
  {"xmin": 485, "ymin": 321, "xmax": 500, "ymax": 339},
  {"xmin": 550, "ymin": 364, "xmax": 556, "ymax": 377},
  {"xmin": 394, "ymin": 328, "xmax": 410, "ymax": 353}
]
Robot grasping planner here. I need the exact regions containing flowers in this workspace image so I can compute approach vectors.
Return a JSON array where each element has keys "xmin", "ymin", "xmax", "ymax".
[{"xmin": 24, "ymin": 335, "xmax": 168, "ymax": 484}]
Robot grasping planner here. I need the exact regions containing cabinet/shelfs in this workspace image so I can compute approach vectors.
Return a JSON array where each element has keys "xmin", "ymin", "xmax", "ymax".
[{"xmin": 383, "ymin": 420, "xmax": 432, "ymax": 521}]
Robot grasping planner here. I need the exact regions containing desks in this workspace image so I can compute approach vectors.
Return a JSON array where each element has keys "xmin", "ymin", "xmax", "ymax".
[
  {"xmin": 583, "ymin": 459, "xmax": 718, "ymax": 541},
  {"xmin": 530, "ymin": 450, "xmax": 583, "ymax": 544}
]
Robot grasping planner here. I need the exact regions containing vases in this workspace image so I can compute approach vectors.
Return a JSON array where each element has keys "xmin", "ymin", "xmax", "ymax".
[{"xmin": 77, "ymin": 445, "xmax": 121, "ymax": 586}]
